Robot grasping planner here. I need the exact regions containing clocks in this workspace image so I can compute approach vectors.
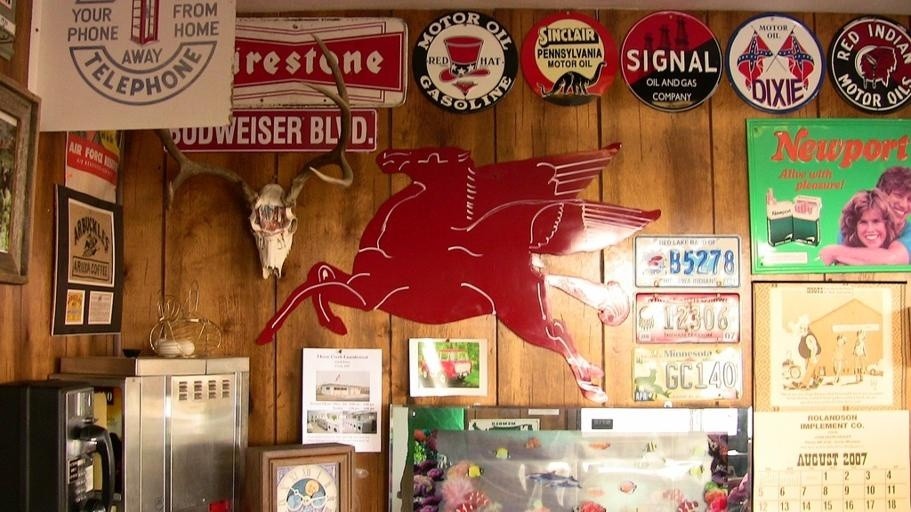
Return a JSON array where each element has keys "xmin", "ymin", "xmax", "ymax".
[{"xmin": 243, "ymin": 440, "xmax": 358, "ymax": 512}]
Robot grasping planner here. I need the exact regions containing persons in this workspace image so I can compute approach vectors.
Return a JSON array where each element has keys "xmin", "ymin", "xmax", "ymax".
[
  {"xmin": 822, "ymin": 189, "xmax": 911, "ymax": 268},
  {"xmin": 841, "ymin": 164, "xmax": 911, "ymax": 248}
]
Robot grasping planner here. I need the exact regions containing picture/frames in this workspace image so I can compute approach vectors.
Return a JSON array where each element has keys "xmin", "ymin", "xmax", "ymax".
[{"xmin": 0, "ymin": 73, "xmax": 43, "ymax": 290}]
